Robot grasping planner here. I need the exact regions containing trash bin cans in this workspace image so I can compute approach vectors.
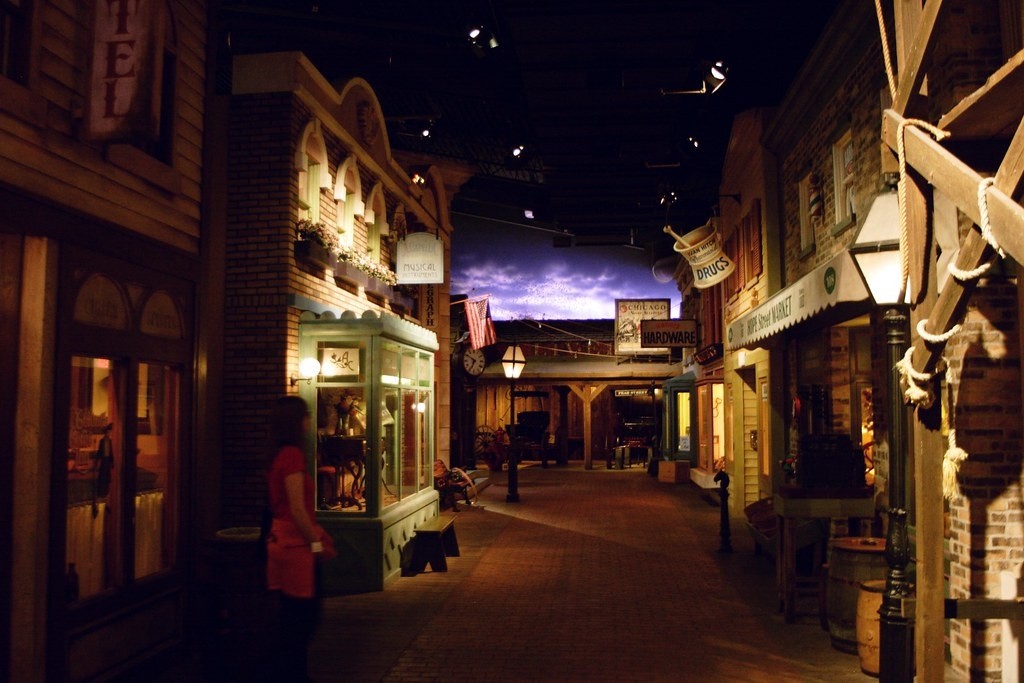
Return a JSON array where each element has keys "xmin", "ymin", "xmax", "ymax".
[
  {"xmin": 675, "ymin": 460, "xmax": 690, "ymax": 484},
  {"xmin": 744, "ymin": 496, "xmax": 819, "ymax": 581}
]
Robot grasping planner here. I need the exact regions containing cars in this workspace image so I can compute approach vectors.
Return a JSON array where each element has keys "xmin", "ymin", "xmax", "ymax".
[{"xmin": 613, "ymin": 421, "xmax": 655, "ymax": 459}]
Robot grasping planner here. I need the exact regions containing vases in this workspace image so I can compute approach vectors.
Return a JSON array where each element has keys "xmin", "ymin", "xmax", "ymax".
[
  {"xmin": 342, "ymin": 414, "xmax": 350, "ymax": 435},
  {"xmin": 334, "ymin": 262, "xmax": 369, "ymax": 288},
  {"xmin": 294, "ymin": 239, "xmax": 338, "ymax": 271},
  {"xmin": 391, "ymin": 292, "xmax": 412, "ymax": 312},
  {"xmin": 365, "ymin": 278, "xmax": 394, "ymax": 300}
]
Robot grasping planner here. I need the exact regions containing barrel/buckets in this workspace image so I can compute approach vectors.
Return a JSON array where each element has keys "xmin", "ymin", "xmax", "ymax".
[
  {"xmin": 855, "ymin": 579, "xmax": 916, "ymax": 678},
  {"xmin": 826, "ymin": 537, "xmax": 887, "ymax": 654}
]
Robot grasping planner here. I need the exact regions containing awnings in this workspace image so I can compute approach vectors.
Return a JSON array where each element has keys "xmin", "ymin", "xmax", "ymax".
[{"xmin": 721, "ymin": 250, "xmax": 868, "ymax": 349}]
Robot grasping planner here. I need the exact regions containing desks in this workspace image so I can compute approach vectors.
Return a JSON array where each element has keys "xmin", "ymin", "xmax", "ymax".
[{"xmin": 772, "ymin": 475, "xmax": 876, "ymax": 622}]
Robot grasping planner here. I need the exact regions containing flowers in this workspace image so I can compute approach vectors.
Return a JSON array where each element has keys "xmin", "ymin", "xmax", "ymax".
[
  {"xmin": 296, "ymin": 217, "xmax": 397, "ymax": 286},
  {"xmin": 398, "ymin": 284, "xmax": 417, "ymax": 300},
  {"xmin": 327, "ymin": 388, "xmax": 365, "ymax": 415}
]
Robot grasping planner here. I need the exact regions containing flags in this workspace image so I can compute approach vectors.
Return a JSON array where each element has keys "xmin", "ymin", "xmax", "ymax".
[{"xmin": 464, "ymin": 297, "xmax": 497, "ymax": 351}]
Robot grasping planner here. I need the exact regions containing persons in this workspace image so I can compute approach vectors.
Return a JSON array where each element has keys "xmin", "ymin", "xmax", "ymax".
[
  {"xmin": 97, "ymin": 428, "xmax": 114, "ymax": 482},
  {"xmin": 259, "ymin": 394, "xmax": 335, "ymax": 678}
]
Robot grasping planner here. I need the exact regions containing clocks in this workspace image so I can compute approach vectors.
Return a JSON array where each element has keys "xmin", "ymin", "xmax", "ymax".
[{"xmin": 463, "ymin": 348, "xmax": 486, "ymax": 376}]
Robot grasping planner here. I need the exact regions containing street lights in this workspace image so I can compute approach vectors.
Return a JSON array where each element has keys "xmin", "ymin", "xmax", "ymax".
[
  {"xmin": 500, "ymin": 340, "xmax": 526, "ymax": 501},
  {"xmin": 850, "ymin": 173, "xmax": 917, "ymax": 683},
  {"xmin": 649, "ymin": 383, "xmax": 661, "ymax": 476}
]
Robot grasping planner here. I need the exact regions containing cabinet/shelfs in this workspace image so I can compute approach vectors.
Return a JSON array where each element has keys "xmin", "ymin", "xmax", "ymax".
[{"xmin": 321, "ymin": 432, "xmax": 394, "ymax": 505}]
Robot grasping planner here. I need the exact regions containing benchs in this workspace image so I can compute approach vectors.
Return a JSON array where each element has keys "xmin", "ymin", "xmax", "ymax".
[
  {"xmin": 412, "ymin": 514, "xmax": 461, "ymax": 573},
  {"xmin": 655, "ymin": 459, "xmax": 690, "ymax": 483}
]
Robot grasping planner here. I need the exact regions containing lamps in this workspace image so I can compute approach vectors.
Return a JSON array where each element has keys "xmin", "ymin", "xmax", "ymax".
[
  {"xmin": 657, "ymin": 58, "xmax": 730, "ymax": 98},
  {"xmin": 290, "ymin": 357, "xmax": 320, "ymax": 387},
  {"xmin": 654, "ymin": 189, "xmax": 679, "ymax": 206},
  {"xmin": 688, "ymin": 130, "xmax": 704, "ymax": 153},
  {"xmin": 456, "ymin": 195, "xmax": 536, "ymax": 220},
  {"xmin": 385, "ymin": 113, "xmax": 433, "ymax": 139}
]
